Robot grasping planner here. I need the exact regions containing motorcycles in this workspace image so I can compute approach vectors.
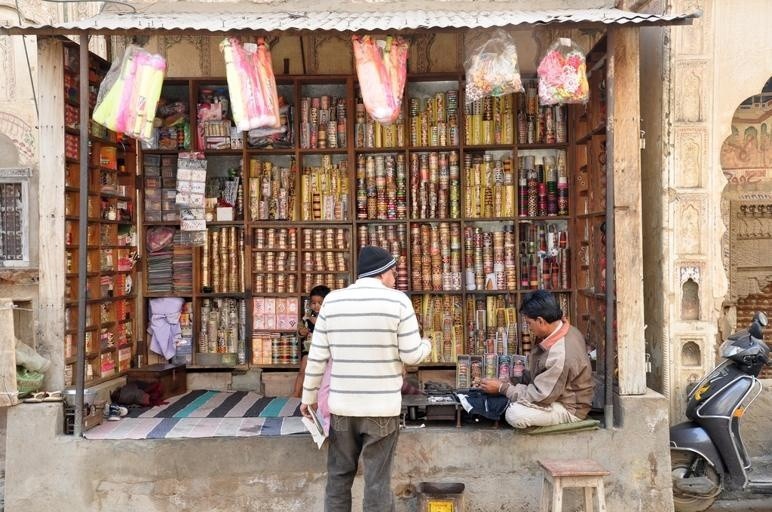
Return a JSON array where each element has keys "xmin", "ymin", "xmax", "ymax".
[{"xmin": 666, "ymin": 309, "xmax": 772, "ymax": 511}]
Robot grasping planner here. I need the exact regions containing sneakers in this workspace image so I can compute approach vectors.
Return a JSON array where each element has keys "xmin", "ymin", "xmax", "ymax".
[
  {"xmin": 104, "ymin": 404, "xmax": 128, "ymax": 416},
  {"xmin": 108, "ymin": 405, "xmax": 121, "ymax": 420}
]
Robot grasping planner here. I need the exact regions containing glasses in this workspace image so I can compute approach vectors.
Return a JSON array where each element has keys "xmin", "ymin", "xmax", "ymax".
[{"xmin": 390, "ymin": 268, "xmax": 398, "ymax": 278}]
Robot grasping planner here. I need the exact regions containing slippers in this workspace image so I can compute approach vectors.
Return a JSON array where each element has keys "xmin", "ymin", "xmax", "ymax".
[
  {"xmin": 42, "ymin": 391, "xmax": 65, "ymax": 401},
  {"xmin": 23, "ymin": 392, "xmax": 45, "ymax": 403}
]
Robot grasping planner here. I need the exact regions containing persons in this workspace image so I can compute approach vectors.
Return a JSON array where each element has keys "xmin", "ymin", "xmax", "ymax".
[
  {"xmin": 289, "ymin": 284, "xmax": 330, "ymax": 398},
  {"xmin": 300, "ymin": 244, "xmax": 432, "ymax": 511},
  {"xmin": 478, "ymin": 289, "xmax": 593, "ymax": 429}
]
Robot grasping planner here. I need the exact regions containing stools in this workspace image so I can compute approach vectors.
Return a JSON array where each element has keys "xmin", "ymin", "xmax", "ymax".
[{"xmin": 537, "ymin": 459, "xmax": 610, "ymax": 512}]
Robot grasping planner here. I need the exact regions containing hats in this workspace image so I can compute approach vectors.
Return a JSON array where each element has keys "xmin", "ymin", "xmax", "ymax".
[{"xmin": 357, "ymin": 246, "xmax": 396, "ymax": 279}]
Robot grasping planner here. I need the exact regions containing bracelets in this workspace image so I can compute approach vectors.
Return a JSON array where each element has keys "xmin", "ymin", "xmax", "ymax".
[{"xmin": 499, "ymin": 382, "xmax": 503, "ymax": 393}]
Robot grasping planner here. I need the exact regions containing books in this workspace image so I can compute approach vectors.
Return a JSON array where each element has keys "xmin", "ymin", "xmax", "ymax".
[{"xmin": 302, "ymin": 404, "xmax": 329, "ymax": 449}]
